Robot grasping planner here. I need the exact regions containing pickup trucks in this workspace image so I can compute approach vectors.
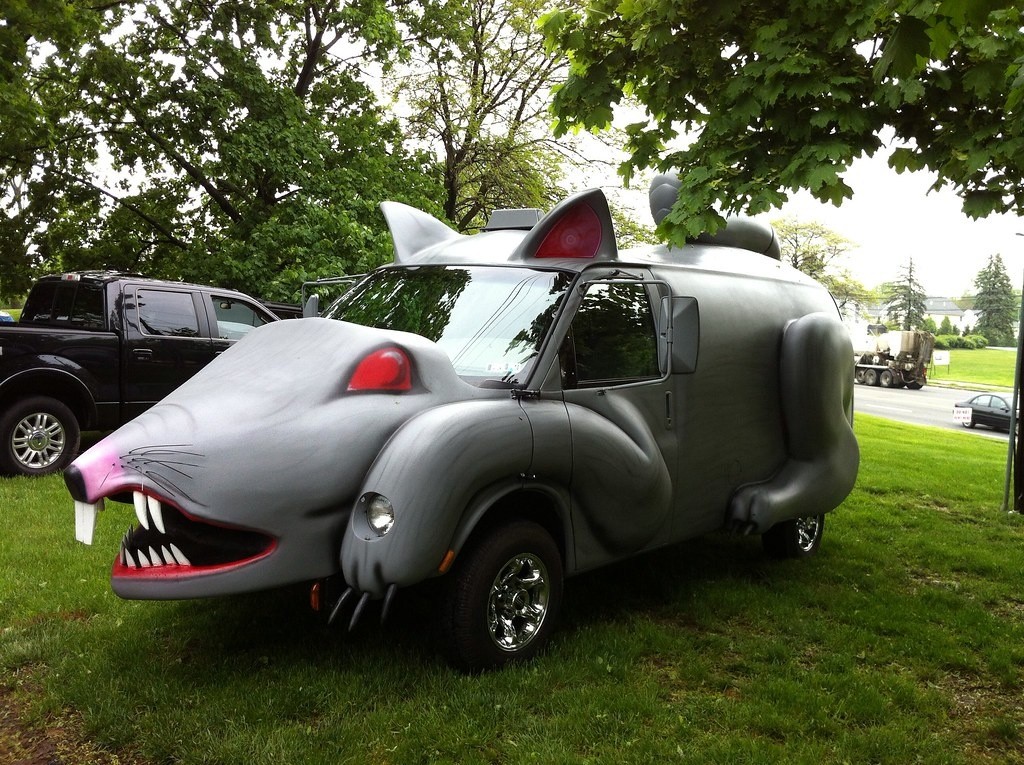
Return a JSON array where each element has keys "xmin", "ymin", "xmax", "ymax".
[{"xmin": 0, "ymin": 269, "xmax": 289, "ymax": 476}]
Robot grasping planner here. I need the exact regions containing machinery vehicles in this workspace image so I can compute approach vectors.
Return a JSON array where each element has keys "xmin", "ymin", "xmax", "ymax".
[{"xmin": 850, "ymin": 318, "xmax": 935, "ymax": 391}]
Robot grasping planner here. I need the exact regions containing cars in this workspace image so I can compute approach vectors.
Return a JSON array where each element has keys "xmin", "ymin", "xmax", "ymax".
[
  {"xmin": 952, "ymin": 392, "xmax": 1020, "ymax": 435},
  {"xmin": 263, "ymin": 302, "xmax": 323, "ymax": 326}
]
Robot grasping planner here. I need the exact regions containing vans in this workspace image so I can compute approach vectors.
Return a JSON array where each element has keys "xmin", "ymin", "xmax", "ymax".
[{"xmin": 61, "ymin": 176, "xmax": 868, "ymax": 666}]
